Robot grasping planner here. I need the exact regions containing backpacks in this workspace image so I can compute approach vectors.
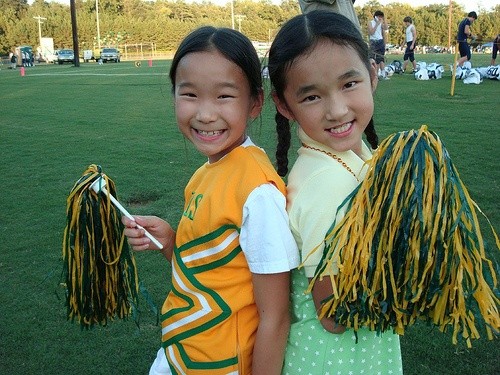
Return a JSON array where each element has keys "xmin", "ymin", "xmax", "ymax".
[{"xmin": 11, "ymin": 56, "xmax": 16, "ymax": 63}]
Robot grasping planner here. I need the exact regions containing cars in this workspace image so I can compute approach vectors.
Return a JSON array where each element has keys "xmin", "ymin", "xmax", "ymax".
[
  {"xmin": 99, "ymin": 49, "xmax": 122, "ymax": 63},
  {"xmin": 55, "ymin": 49, "xmax": 75, "ymax": 65},
  {"xmin": 480, "ymin": 42, "xmax": 493, "ymax": 53}
]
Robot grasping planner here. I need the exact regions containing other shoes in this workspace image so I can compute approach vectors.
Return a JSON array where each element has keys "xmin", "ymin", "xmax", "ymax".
[{"xmin": 381, "ymin": 76, "xmax": 390, "ymax": 80}]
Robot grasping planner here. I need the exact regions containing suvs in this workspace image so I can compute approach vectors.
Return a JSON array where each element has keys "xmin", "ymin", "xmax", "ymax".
[{"xmin": 19, "ymin": 46, "xmax": 35, "ymax": 63}]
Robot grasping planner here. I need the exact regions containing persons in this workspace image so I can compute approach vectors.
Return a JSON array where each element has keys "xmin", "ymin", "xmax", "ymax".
[
  {"xmin": 491, "ymin": 36, "xmax": 500, "ymax": 66},
  {"xmin": 21, "ymin": 50, "xmax": 34, "ymax": 67},
  {"xmin": 400, "ymin": 17, "xmax": 416, "ymax": 72},
  {"xmin": 10, "ymin": 54, "xmax": 17, "ymax": 70},
  {"xmin": 469, "ymin": 44, "xmax": 496, "ymax": 54},
  {"xmin": 262, "ymin": 10, "xmax": 404, "ymax": 375},
  {"xmin": 415, "ymin": 44, "xmax": 455, "ymax": 54},
  {"xmin": 38, "ymin": 53, "xmax": 44, "ymax": 63},
  {"xmin": 383, "ymin": 44, "xmax": 407, "ymax": 55},
  {"xmin": 368, "ymin": 11, "xmax": 390, "ymax": 80},
  {"xmin": 121, "ymin": 26, "xmax": 301, "ymax": 375},
  {"xmin": 456, "ymin": 11, "xmax": 478, "ymax": 67}
]
